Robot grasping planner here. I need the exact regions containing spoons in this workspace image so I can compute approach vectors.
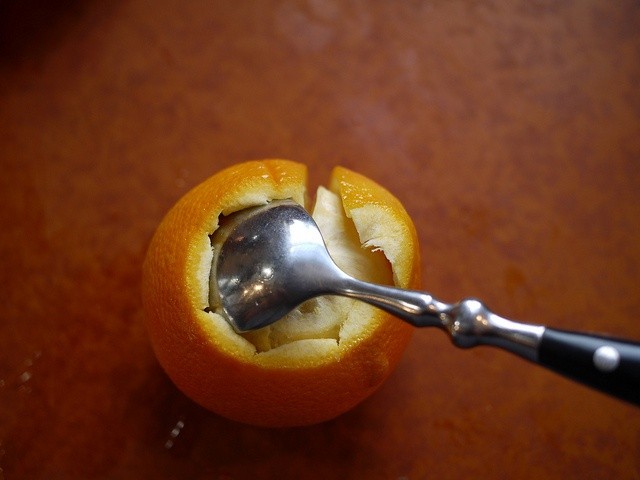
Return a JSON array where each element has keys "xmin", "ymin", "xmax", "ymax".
[{"xmin": 206, "ymin": 198, "xmax": 640, "ymax": 410}]
